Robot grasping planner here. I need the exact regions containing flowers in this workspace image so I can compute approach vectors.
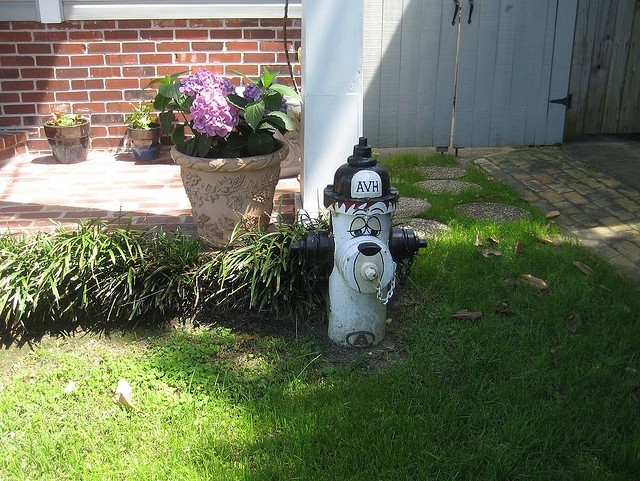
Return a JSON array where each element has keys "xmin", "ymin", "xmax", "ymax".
[{"xmin": 143, "ymin": 66, "xmax": 303, "ymax": 158}]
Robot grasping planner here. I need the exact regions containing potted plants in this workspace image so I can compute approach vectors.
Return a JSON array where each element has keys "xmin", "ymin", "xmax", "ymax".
[
  {"xmin": 43, "ymin": 111, "xmax": 90, "ymax": 164},
  {"xmin": 124, "ymin": 99, "xmax": 162, "ymax": 161}
]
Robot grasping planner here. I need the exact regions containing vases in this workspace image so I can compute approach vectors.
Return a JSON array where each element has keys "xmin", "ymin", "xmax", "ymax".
[{"xmin": 170, "ymin": 138, "xmax": 289, "ymax": 249}]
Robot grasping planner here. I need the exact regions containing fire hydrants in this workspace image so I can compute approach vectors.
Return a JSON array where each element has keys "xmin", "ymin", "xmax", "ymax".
[{"xmin": 286, "ymin": 136, "xmax": 431, "ymax": 351}]
